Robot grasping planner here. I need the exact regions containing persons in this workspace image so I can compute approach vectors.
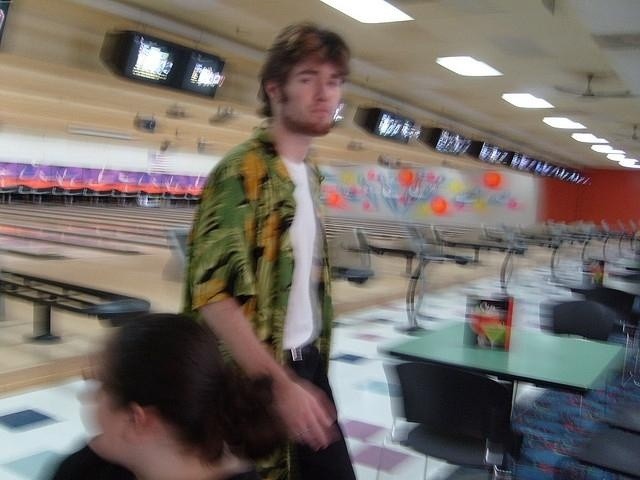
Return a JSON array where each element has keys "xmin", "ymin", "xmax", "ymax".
[
  {"xmin": 35, "ymin": 313, "xmax": 287, "ymax": 480},
  {"xmin": 178, "ymin": 19, "xmax": 359, "ymax": 480}
]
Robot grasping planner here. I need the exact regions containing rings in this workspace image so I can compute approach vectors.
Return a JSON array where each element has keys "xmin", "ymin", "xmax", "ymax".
[{"xmin": 296, "ymin": 429, "xmax": 310, "ymax": 438}]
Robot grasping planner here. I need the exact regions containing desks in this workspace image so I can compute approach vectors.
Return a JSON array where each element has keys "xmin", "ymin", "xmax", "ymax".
[{"xmin": 387, "ymin": 322, "xmax": 624, "ymax": 427}]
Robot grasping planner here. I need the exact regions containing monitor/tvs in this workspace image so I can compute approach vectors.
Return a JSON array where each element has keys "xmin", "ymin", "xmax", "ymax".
[
  {"xmin": 391, "ymin": 115, "xmax": 414, "ymax": 143},
  {"xmin": 99, "ymin": 30, "xmax": 182, "ymax": 87},
  {"xmin": 170, "ymin": 44, "xmax": 225, "ymax": 97},
  {"xmin": 425, "ymin": 128, "xmax": 473, "ymax": 157},
  {"xmin": 467, "ymin": 140, "xmax": 591, "ymax": 185},
  {"xmin": 360, "ymin": 108, "xmax": 399, "ymax": 139}
]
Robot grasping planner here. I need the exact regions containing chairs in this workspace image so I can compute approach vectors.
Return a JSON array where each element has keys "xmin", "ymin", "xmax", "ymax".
[{"xmin": 399, "ymin": 283, "xmax": 639, "ymax": 480}]
